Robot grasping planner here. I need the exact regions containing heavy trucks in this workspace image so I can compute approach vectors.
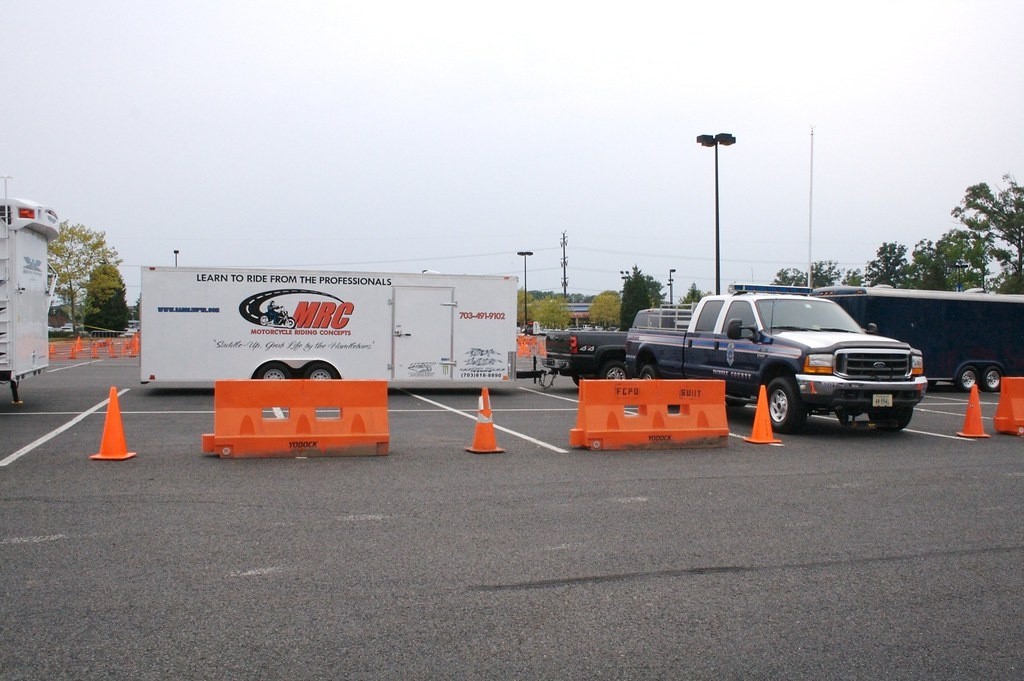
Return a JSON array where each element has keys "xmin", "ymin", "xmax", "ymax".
[{"xmin": 810, "ymin": 284, "xmax": 1024, "ymax": 393}]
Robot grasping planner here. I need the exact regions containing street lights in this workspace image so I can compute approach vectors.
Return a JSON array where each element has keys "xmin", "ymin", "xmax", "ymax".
[
  {"xmin": 670, "ymin": 269, "xmax": 676, "ymax": 304},
  {"xmin": 620, "ymin": 271, "xmax": 629, "ymax": 283},
  {"xmin": 697, "ymin": 133, "xmax": 736, "ymax": 295},
  {"xmin": 517, "ymin": 252, "xmax": 533, "ymax": 335},
  {"xmin": 952, "ymin": 264, "xmax": 968, "ymax": 292},
  {"xmin": 173, "ymin": 250, "xmax": 179, "ymax": 267}
]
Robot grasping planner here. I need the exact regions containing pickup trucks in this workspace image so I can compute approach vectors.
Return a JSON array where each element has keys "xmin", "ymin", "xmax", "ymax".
[
  {"xmin": 625, "ymin": 292, "xmax": 929, "ymax": 432},
  {"xmin": 541, "ymin": 307, "xmax": 692, "ymax": 392}
]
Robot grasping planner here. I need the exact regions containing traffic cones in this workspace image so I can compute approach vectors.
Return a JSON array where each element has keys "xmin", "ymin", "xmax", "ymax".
[
  {"xmin": 464, "ymin": 386, "xmax": 505, "ymax": 453},
  {"xmin": 742, "ymin": 385, "xmax": 782, "ymax": 444},
  {"xmin": 71, "ymin": 336, "xmax": 141, "ymax": 359},
  {"xmin": 89, "ymin": 386, "xmax": 136, "ymax": 460},
  {"xmin": 49, "ymin": 344, "xmax": 57, "ymax": 354},
  {"xmin": 957, "ymin": 385, "xmax": 991, "ymax": 438}
]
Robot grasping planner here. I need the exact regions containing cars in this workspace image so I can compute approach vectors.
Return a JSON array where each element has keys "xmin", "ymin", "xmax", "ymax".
[{"xmin": 583, "ymin": 324, "xmax": 617, "ymax": 331}]
